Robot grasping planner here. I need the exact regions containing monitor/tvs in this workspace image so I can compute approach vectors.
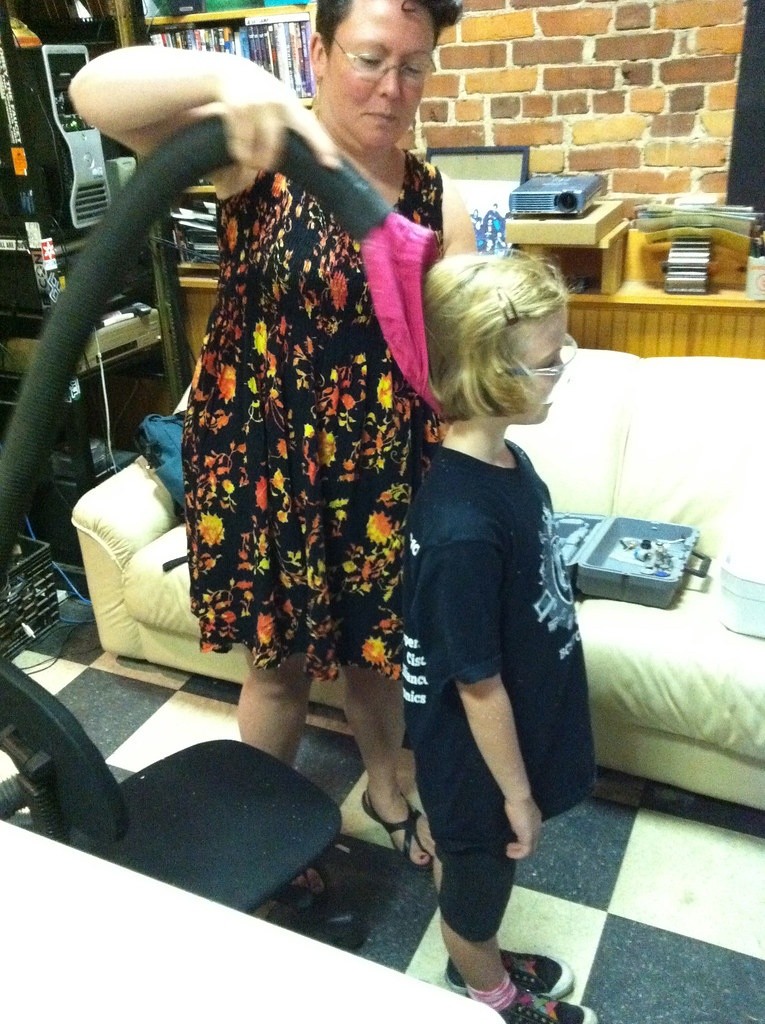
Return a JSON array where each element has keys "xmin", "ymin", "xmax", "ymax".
[{"xmin": 425, "ymin": 147, "xmax": 529, "ymax": 219}]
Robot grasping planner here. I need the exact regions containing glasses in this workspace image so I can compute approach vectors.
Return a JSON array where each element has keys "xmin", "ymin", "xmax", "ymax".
[
  {"xmin": 332, "ymin": 37, "xmax": 437, "ymax": 86},
  {"xmin": 513, "ymin": 331, "xmax": 579, "ymax": 405}
]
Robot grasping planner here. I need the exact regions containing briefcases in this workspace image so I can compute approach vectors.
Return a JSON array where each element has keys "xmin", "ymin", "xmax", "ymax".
[{"xmin": 551, "ymin": 511, "xmax": 711, "ymax": 608}]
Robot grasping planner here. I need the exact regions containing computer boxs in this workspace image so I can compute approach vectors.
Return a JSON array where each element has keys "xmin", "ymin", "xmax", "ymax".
[{"xmin": 0, "ymin": 45, "xmax": 113, "ymax": 238}]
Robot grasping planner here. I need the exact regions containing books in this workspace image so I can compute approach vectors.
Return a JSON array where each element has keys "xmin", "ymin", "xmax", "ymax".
[
  {"xmin": 144, "ymin": 22, "xmax": 320, "ymax": 102},
  {"xmin": 632, "ymin": 204, "xmax": 763, "ymax": 293}
]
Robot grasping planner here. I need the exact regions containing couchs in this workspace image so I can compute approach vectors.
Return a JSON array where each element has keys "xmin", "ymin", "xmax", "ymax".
[{"xmin": 72, "ymin": 342, "xmax": 764, "ymax": 809}]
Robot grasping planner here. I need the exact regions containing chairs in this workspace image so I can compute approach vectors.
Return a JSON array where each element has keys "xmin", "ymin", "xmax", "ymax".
[{"xmin": 0, "ymin": 657, "xmax": 364, "ymax": 947}]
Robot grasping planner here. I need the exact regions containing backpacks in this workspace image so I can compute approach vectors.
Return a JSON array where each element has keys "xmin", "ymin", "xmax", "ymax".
[{"xmin": 130, "ymin": 409, "xmax": 187, "ymax": 520}]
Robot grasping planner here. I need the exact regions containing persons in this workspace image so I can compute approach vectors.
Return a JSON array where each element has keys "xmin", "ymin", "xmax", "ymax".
[
  {"xmin": 66, "ymin": 1, "xmax": 468, "ymax": 900},
  {"xmin": 397, "ymin": 249, "xmax": 603, "ymax": 1024}
]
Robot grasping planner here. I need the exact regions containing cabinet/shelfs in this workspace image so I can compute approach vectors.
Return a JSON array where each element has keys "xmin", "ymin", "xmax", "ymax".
[{"xmin": 139, "ymin": 1, "xmax": 320, "ymax": 388}]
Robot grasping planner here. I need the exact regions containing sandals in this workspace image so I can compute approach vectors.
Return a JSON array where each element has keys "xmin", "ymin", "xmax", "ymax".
[
  {"xmin": 362, "ymin": 788, "xmax": 434, "ymax": 872},
  {"xmin": 280, "ymin": 859, "xmax": 331, "ymax": 912}
]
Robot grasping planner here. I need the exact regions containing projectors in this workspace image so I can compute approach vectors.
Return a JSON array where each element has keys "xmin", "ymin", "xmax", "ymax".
[{"xmin": 509, "ymin": 177, "xmax": 601, "ymax": 219}]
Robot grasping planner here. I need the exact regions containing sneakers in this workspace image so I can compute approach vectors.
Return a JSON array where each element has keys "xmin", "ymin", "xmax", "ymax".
[
  {"xmin": 466, "ymin": 984, "xmax": 599, "ymax": 1024},
  {"xmin": 445, "ymin": 947, "xmax": 574, "ymax": 1001}
]
